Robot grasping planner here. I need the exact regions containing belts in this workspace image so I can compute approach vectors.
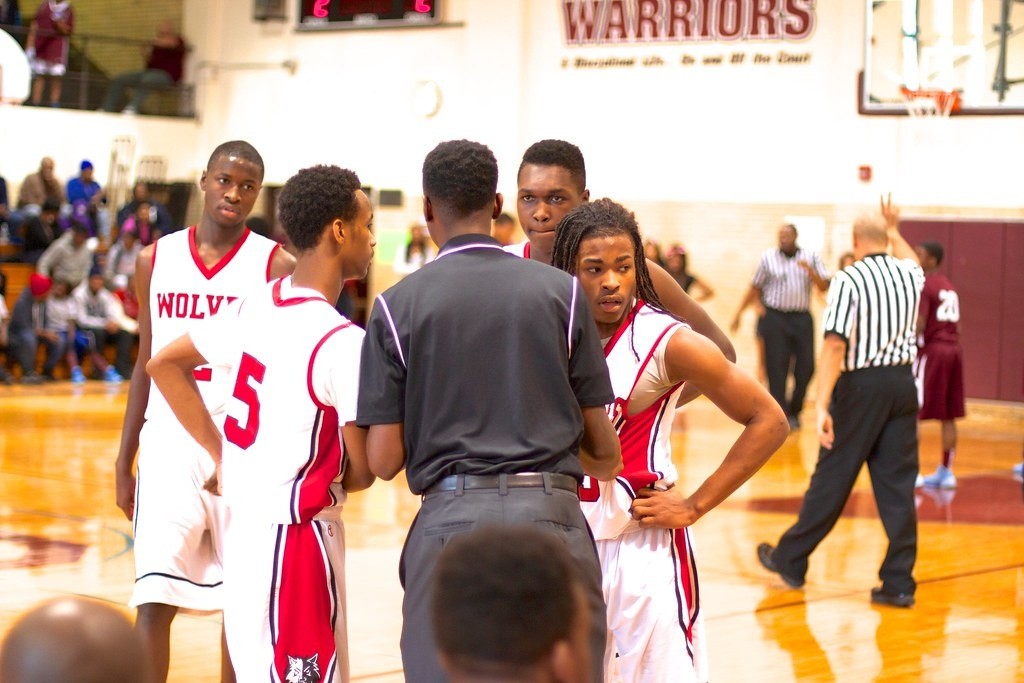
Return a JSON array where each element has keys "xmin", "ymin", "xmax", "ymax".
[{"xmin": 422, "ymin": 473, "xmax": 578, "ymax": 493}]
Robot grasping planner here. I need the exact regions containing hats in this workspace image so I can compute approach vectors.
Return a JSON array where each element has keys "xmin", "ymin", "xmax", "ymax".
[
  {"xmin": 122, "ymin": 217, "xmax": 136, "ymax": 233},
  {"xmin": 30, "ymin": 273, "xmax": 53, "ymax": 298},
  {"xmin": 81, "ymin": 161, "xmax": 93, "ymax": 169}
]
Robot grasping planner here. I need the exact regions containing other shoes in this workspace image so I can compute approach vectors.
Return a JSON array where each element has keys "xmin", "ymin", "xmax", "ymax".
[
  {"xmin": 922, "ymin": 485, "xmax": 956, "ymax": 504},
  {"xmin": 927, "ymin": 467, "xmax": 955, "ymax": 489},
  {"xmin": 787, "ymin": 417, "xmax": 801, "ymax": 434},
  {"xmin": 758, "ymin": 542, "xmax": 805, "ymax": 588},
  {"xmin": 914, "ymin": 473, "xmax": 926, "ymax": 486},
  {"xmin": 870, "ymin": 583, "xmax": 913, "ymax": 606},
  {"xmin": 102, "ymin": 365, "xmax": 123, "ymax": 382},
  {"xmin": 71, "ymin": 366, "xmax": 86, "ymax": 381}
]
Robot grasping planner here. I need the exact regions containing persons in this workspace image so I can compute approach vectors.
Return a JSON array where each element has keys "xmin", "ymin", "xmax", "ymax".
[
  {"xmin": 551, "ymin": 196, "xmax": 790, "ymax": 683},
  {"xmin": 429, "ymin": 523, "xmax": 604, "ymax": 683},
  {"xmin": 111, "ymin": 177, "xmax": 177, "ymax": 247},
  {"xmin": 0, "ymin": 159, "xmax": 107, "ymax": 262},
  {"xmin": 24, "ymin": 0, "xmax": 77, "ymax": 108},
  {"xmin": 394, "ymin": 224, "xmax": 435, "ymax": 275},
  {"xmin": 757, "ymin": 190, "xmax": 926, "ymax": 608},
  {"xmin": 489, "ymin": 213, "xmax": 513, "ymax": 246},
  {"xmin": 0, "ymin": 231, "xmax": 169, "ymax": 385},
  {"xmin": 646, "ymin": 240, "xmax": 714, "ymax": 304},
  {"xmin": 0, "ymin": 593, "xmax": 163, "ymax": 683},
  {"xmin": 95, "ymin": 16, "xmax": 188, "ymax": 116},
  {"xmin": 499, "ymin": 139, "xmax": 738, "ymax": 408},
  {"xmin": 144, "ymin": 163, "xmax": 374, "ymax": 683},
  {"xmin": 731, "ymin": 223, "xmax": 833, "ymax": 430},
  {"xmin": 355, "ymin": 140, "xmax": 626, "ymax": 683},
  {"xmin": 911, "ymin": 239, "xmax": 966, "ymax": 489},
  {"xmin": 836, "ymin": 253, "xmax": 855, "ymax": 269},
  {"xmin": 115, "ymin": 140, "xmax": 294, "ymax": 683}
]
